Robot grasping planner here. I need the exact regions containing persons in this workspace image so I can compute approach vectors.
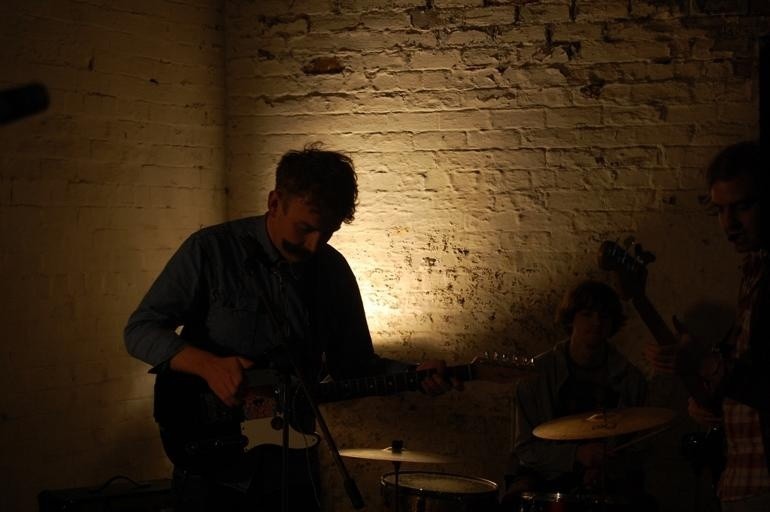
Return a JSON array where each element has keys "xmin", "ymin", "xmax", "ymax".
[
  {"xmin": 639, "ymin": 136, "xmax": 767, "ymax": 509},
  {"xmin": 122, "ymin": 138, "xmax": 466, "ymax": 511},
  {"xmin": 497, "ymin": 274, "xmax": 647, "ymax": 511}
]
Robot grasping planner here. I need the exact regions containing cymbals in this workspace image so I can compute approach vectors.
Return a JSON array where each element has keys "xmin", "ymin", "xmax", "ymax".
[
  {"xmin": 336, "ymin": 447, "xmax": 458, "ymax": 465},
  {"xmin": 533, "ymin": 406, "xmax": 678, "ymax": 441}
]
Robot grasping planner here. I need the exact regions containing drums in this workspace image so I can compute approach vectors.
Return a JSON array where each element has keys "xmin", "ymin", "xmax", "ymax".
[
  {"xmin": 380, "ymin": 471, "xmax": 499, "ymax": 512},
  {"xmin": 519, "ymin": 491, "xmax": 608, "ymax": 512}
]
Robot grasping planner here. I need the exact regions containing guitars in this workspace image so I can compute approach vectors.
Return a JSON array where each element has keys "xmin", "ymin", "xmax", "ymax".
[
  {"xmin": 597, "ymin": 236, "xmax": 731, "ymax": 448},
  {"xmin": 153, "ymin": 342, "xmax": 542, "ymax": 479}
]
